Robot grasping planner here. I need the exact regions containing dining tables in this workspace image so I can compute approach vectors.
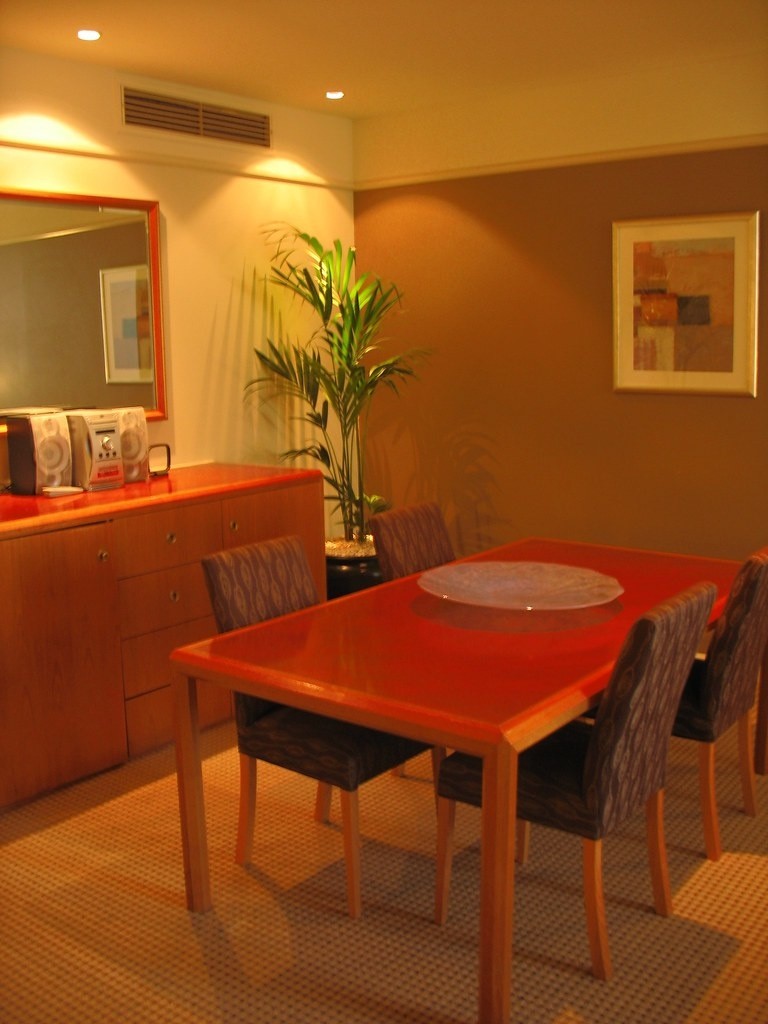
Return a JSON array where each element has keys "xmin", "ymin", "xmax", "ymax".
[{"xmin": 166, "ymin": 538, "xmax": 768, "ymax": 1024}]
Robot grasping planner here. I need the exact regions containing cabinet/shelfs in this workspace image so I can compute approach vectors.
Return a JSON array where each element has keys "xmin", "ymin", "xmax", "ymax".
[{"xmin": 1, "ymin": 462, "xmax": 327, "ymax": 814}]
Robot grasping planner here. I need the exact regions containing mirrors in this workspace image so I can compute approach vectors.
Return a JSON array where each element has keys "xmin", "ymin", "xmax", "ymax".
[{"xmin": 0, "ymin": 186, "xmax": 167, "ymax": 436}]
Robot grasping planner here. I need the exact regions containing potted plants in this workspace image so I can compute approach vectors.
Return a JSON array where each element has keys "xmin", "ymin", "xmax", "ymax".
[{"xmin": 242, "ymin": 223, "xmax": 436, "ymax": 601}]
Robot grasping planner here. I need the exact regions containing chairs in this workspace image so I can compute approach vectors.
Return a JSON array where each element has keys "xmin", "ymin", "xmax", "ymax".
[
  {"xmin": 580, "ymin": 543, "xmax": 768, "ymax": 863},
  {"xmin": 366, "ymin": 500, "xmax": 458, "ymax": 778},
  {"xmin": 433, "ymin": 581, "xmax": 718, "ymax": 981},
  {"xmin": 200, "ymin": 536, "xmax": 449, "ymax": 921}
]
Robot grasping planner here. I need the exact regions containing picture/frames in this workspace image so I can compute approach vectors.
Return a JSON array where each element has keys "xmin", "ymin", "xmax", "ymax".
[
  {"xmin": 99, "ymin": 265, "xmax": 153, "ymax": 385},
  {"xmin": 611, "ymin": 211, "xmax": 758, "ymax": 398}
]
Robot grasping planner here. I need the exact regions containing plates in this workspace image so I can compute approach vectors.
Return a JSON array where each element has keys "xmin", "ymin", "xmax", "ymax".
[{"xmin": 417, "ymin": 561, "xmax": 623, "ymax": 609}]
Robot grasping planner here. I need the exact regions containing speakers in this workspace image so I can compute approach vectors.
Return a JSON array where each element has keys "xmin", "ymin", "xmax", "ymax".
[
  {"xmin": 7, "ymin": 413, "xmax": 73, "ymax": 495},
  {"xmin": 110, "ymin": 405, "xmax": 148, "ymax": 483}
]
